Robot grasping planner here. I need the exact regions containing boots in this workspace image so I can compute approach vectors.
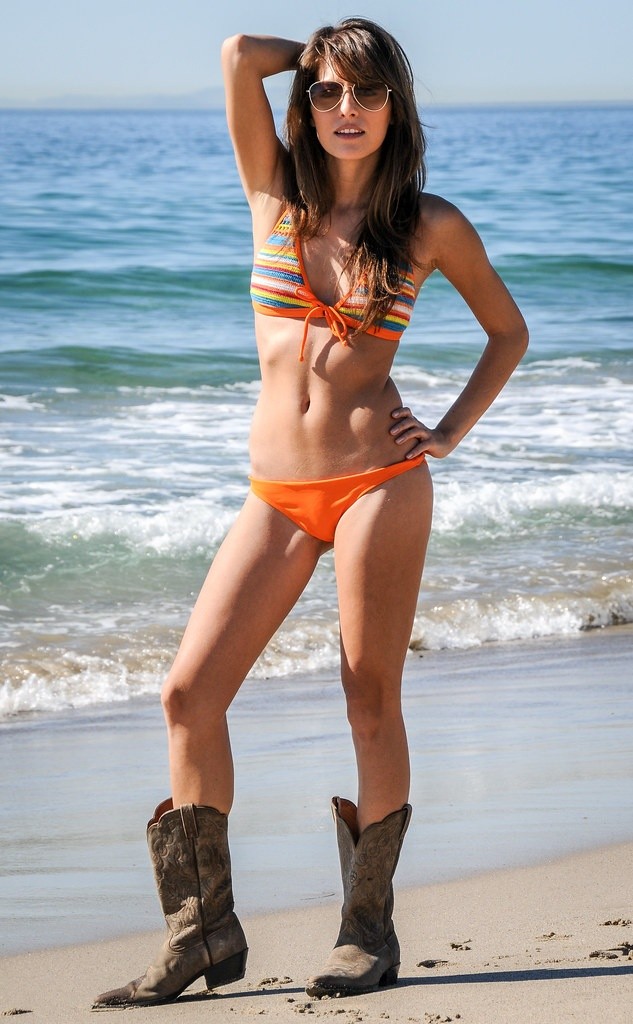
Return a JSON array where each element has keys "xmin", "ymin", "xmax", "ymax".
[
  {"xmin": 93, "ymin": 798, "xmax": 248, "ymax": 1008},
  {"xmin": 304, "ymin": 796, "xmax": 412, "ymax": 997}
]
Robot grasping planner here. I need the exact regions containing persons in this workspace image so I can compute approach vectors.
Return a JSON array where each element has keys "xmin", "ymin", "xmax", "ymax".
[{"xmin": 91, "ymin": 14, "xmax": 531, "ymax": 1009}]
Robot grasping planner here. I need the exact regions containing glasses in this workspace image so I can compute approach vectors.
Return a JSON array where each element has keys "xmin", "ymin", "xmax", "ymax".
[{"xmin": 304, "ymin": 79, "xmax": 392, "ymax": 112}]
